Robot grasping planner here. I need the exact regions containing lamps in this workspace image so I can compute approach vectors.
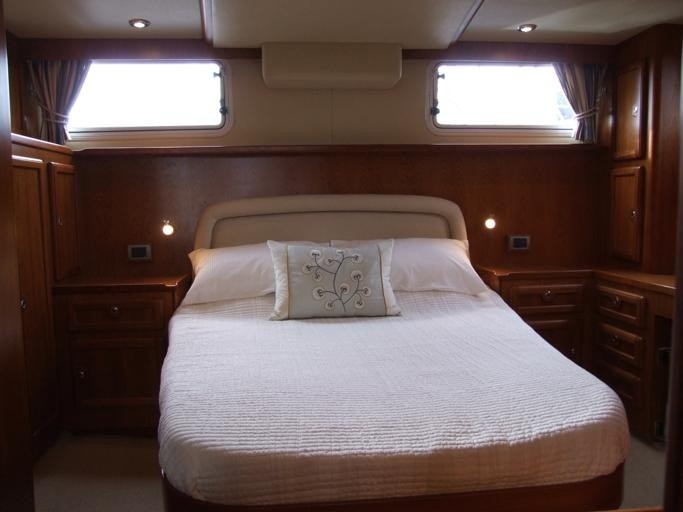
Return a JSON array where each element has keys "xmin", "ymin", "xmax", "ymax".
[
  {"xmin": 129, "ymin": 19, "xmax": 150, "ymax": 29},
  {"xmin": 519, "ymin": 24, "xmax": 536, "ymax": 32},
  {"xmin": 162, "ymin": 223, "xmax": 176, "ymax": 236},
  {"xmin": 485, "ymin": 217, "xmax": 496, "ymax": 229}
]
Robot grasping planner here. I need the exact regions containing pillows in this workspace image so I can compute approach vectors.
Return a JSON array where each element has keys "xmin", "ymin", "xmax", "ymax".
[
  {"xmin": 330, "ymin": 238, "xmax": 488, "ymax": 296},
  {"xmin": 267, "ymin": 238, "xmax": 402, "ymax": 320},
  {"xmin": 181, "ymin": 240, "xmax": 329, "ymax": 306}
]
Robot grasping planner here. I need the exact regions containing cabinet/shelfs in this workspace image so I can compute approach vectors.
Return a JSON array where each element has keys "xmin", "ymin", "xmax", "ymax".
[
  {"xmin": 477, "ymin": 266, "xmax": 647, "ymax": 438},
  {"xmin": 10, "ymin": 155, "xmax": 80, "ymax": 466},
  {"xmin": 598, "ymin": 62, "xmax": 656, "ymax": 259}
]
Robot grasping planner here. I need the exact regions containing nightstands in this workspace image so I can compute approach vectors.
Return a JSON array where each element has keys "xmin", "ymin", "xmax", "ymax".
[
  {"xmin": 159, "ymin": 194, "xmax": 631, "ymax": 512},
  {"xmin": 53, "ymin": 271, "xmax": 192, "ymax": 440}
]
